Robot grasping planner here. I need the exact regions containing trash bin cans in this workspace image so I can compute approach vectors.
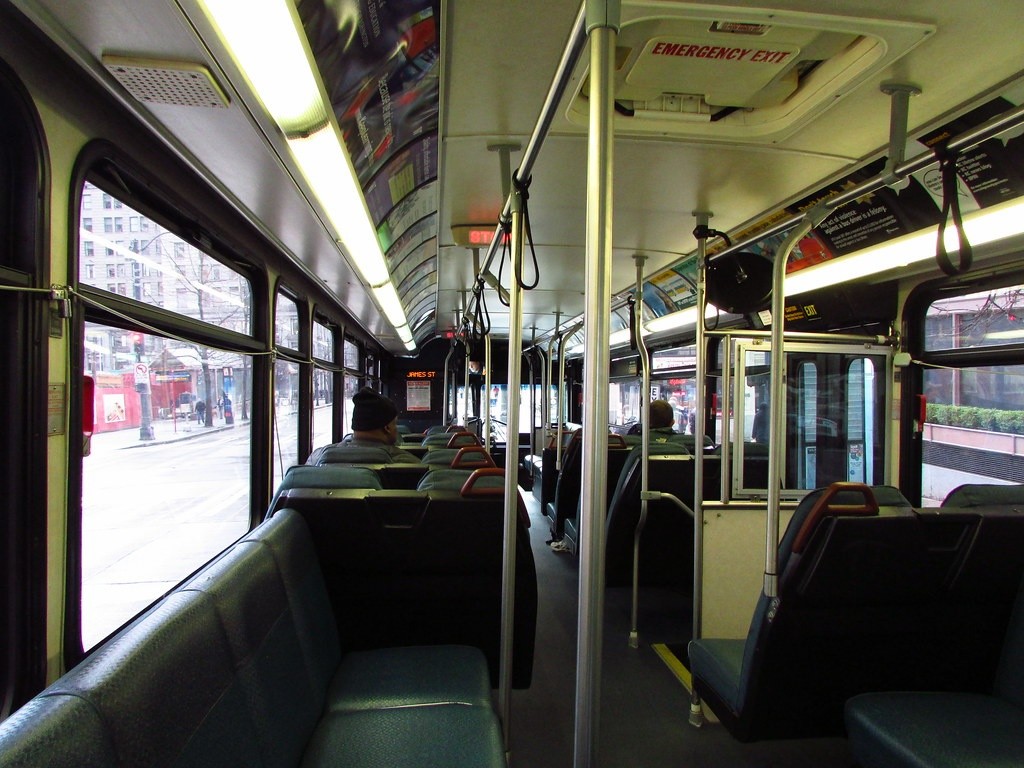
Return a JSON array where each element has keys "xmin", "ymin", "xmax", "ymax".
[{"xmin": 224, "ymin": 404, "xmax": 234, "ymax": 424}]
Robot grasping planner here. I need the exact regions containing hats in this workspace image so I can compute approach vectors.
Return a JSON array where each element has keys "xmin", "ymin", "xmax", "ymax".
[
  {"xmin": 351, "ymin": 386, "xmax": 398, "ymax": 431},
  {"xmin": 197, "ymin": 398, "xmax": 201, "ymax": 402}
]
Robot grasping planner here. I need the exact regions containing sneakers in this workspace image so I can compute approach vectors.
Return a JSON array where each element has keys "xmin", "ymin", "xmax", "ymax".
[{"xmin": 550, "ymin": 540, "xmax": 570, "ymax": 552}]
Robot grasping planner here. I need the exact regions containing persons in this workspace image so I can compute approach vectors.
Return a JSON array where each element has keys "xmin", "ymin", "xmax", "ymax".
[
  {"xmin": 469, "ymin": 361, "xmax": 486, "ymax": 375},
  {"xmin": 195, "ymin": 398, "xmax": 206, "ymax": 425},
  {"xmin": 752, "ymin": 402, "xmax": 767, "ymax": 443},
  {"xmin": 551, "ymin": 401, "xmax": 677, "ymax": 551},
  {"xmin": 304, "ymin": 387, "xmax": 421, "ymax": 466},
  {"xmin": 216, "ymin": 393, "xmax": 231, "ymax": 419},
  {"xmin": 667, "ymin": 397, "xmax": 696, "ymax": 435}
]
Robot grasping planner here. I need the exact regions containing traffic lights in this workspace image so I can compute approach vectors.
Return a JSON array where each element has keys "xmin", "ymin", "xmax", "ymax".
[{"xmin": 133, "ymin": 331, "xmax": 144, "ymax": 353}]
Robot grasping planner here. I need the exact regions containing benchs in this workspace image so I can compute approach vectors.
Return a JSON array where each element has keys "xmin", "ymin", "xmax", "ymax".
[{"xmin": 0, "ymin": 424, "xmax": 1024, "ymax": 768}]
